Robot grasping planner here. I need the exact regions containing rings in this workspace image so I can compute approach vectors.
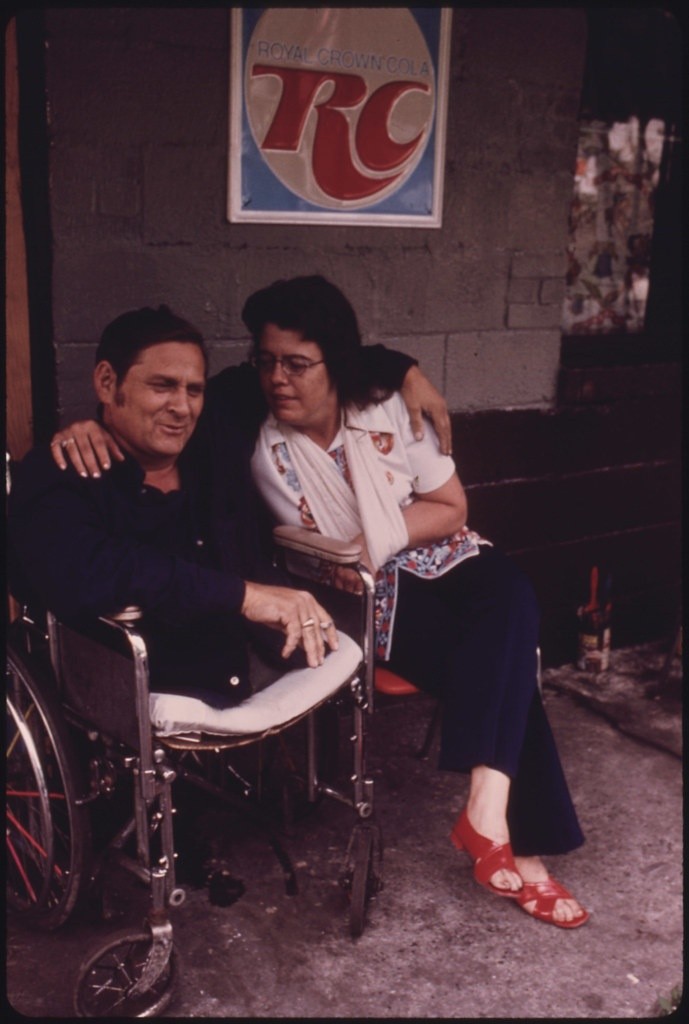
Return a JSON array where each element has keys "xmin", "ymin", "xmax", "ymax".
[
  {"xmin": 50, "ymin": 439, "xmax": 59, "ymax": 448},
  {"xmin": 301, "ymin": 617, "xmax": 315, "ymax": 629},
  {"xmin": 319, "ymin": 620, "xmax": 334, "ymax": 629},
  {"xmin": 60, "ymin": 437, "xmax": 74, "ymax": 447}
]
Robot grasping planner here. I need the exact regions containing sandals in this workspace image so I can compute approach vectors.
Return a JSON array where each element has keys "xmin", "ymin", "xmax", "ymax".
[
  {"xmin": 450, "ymin": 806, "xmax": 525, "ymax": 897},
  {"xmin": 511, "ymin": 861, "xmax": 589, "ymax": 928}
]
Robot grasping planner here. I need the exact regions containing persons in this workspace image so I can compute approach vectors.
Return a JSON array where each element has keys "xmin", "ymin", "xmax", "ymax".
[
  {"xmin": 0, "ymin": 302, "xmax": 453, "ymax": 719},
  {"xmin": 49, "ymin": 270, "xmax": 589, "ymax": 930}
]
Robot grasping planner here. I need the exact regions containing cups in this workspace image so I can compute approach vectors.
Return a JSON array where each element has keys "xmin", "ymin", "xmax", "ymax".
[{"xmin": 575, "ymin": 614, "xmax": 612, "ymax": 674}]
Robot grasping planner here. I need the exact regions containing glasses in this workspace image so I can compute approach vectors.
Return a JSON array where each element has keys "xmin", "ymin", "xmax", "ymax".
[{"xmin": 250, "ymin": 351, "xmax": 328, "ymax": 377}]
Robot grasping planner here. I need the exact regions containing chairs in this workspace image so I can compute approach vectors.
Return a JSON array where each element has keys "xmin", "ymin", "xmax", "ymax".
[{"xmin": 316, "ymin": 666, "xmax": 443, "ymax": 780}]
